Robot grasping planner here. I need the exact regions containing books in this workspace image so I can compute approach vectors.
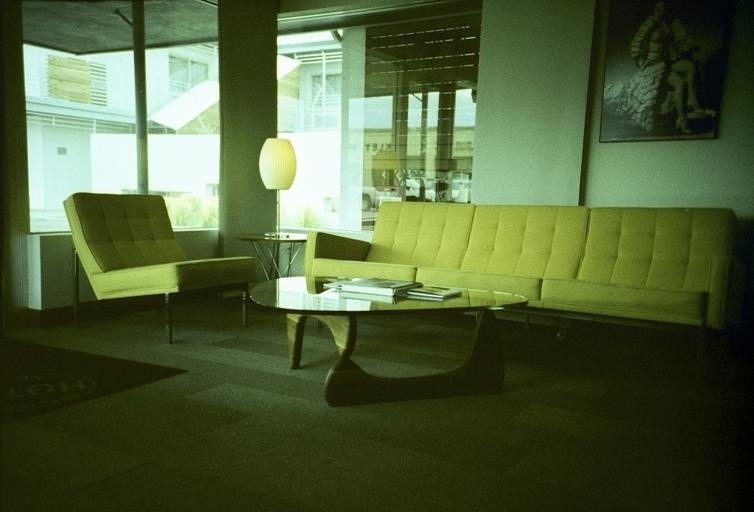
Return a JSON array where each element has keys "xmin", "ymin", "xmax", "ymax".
[{"xmin": 341, "ymin": 278, "xmax": 462, "ymax": 304}]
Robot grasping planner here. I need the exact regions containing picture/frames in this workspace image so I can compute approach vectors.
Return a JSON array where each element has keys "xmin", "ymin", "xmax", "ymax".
[{"xmin": 599, "ymin": 1, "xmax": 733, "ymax": 144}]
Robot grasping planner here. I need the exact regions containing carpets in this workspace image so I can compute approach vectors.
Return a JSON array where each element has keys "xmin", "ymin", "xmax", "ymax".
[{"xmin": 0, "ymin": 334, "xmax": 189, "ymax": 430}]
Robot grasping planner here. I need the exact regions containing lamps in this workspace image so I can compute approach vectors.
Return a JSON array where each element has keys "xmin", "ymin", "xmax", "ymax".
[{"xmin": 259, "ymin": 136, "xmax": 296, "ymax": 238}]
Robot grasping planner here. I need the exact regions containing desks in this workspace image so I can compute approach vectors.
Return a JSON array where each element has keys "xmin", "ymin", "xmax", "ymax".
[{"xmin": 236, "ymin": 232, "xmax": 306, "ymax": 281}]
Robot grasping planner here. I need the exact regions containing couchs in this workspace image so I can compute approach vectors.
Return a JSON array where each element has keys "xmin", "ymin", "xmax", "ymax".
[{"xmin": 305, "ymin": 200, "xmax": 735, "ymax": 376}]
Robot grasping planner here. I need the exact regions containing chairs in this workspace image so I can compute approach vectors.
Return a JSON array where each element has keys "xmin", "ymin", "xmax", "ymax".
[{"xmin": 64, "ymin": 191, "xmax": 254, "ymax": 343}]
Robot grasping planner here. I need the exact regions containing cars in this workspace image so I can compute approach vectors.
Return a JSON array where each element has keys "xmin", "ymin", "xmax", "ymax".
[{"xmin": 363, "ymin": 168, "xmax": 471, "ymax": 214}]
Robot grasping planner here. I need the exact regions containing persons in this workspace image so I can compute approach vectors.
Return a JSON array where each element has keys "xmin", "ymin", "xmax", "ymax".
[{"xmin": 630, "ymin": 1, "xmax": 717, "ymax": 134}]
